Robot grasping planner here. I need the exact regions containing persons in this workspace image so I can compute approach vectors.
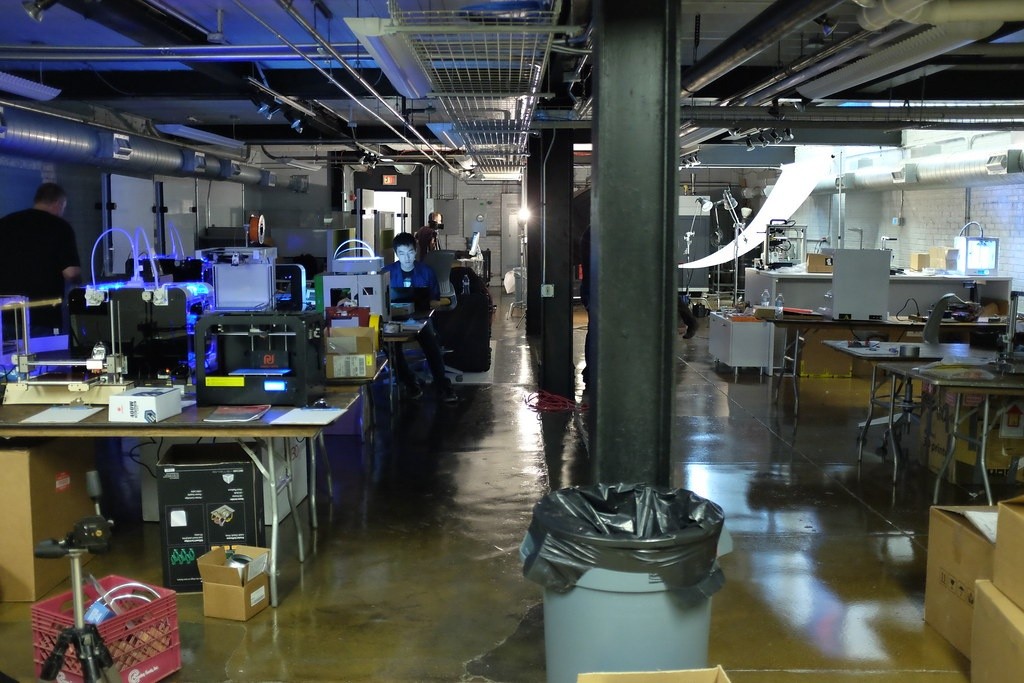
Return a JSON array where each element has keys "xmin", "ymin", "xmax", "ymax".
[
  {"xmin": 415, "ymin": 212, "xmax": 442, "ymax": 252},
  {"xmin": 679, "ymin": 298, "xmax": 699, "ymax": 339},
  {"xmin": 383, "ymin": 231, "xmax": 458, "ymax": 403},
  {"xmin": 580, "ymin": 225, "xmax": 590, "ymax": 376},
  {"xmin": 0, "ymin": 182, "xmax": 80, "ymax": 339}
]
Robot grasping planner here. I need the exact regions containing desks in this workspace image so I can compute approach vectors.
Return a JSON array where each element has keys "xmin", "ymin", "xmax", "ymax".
[
  {"xmin": 764, "ymin": 317, "xmax": 1024, "ymax": 508},
  {"xmin": 0, "ymin": 308, "xmax": 436, "ymax": 606}
]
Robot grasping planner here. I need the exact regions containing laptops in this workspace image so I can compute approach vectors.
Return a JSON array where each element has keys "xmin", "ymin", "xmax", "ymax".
[{"xmin": 390, "ymin": 285, "xmax": 434, "ymax": 311}]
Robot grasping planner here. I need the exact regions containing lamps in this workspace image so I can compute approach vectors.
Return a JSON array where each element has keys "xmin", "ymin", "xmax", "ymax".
[
  {"xmin": 243, "ymin": 81, "xmax": 303, "ymax": 133},
  {"xmin": 463, "ymin": 170, "xmax": 476, "ymax": 178},
  {"xmin": 678, "ymin": 153, "xmax": 701, "ymax": 171},
  {"xmin": 880, "ymin": 236, "xmax": 897, "ymax": 251},
  {"xmin": 849, "ymin": 228, "xmax": 862, "ymax": 250},
  {"xmin": 767, "ymin": 99, "xmax": 785, "ymax": 121},
  {"xmin": 682, "ymin": 190, "xmax": 753, "ymax": 309},
  {"xmin": 959, "ymin": 220, "xmax": 988, "ymax": 249},
  {"xmin": 813, "ymin": 13, "xmax": 839, "ymax": 37},
  {"xmin": 795, "ymin": 98, "xmax": 811, "ymax": 110},
  {"xmin": 343, "ymin": 17, "xmax": 431, "ymax": 100},
  {"xmin": 454, "ymin": 154, "xmax": 476, "ymax": 169},
  {"xmin": 744, "ymin": 126, "xmax": 795, "ymax": 150},
  {"xmin": 425, "ymin": 122, "xmax": 464, "ymax": 149}
]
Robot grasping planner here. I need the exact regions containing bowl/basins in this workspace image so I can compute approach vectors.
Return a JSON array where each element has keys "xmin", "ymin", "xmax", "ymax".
[{"xmin": 951, "ymin": 312, "xmax": 974, "ymax": 322}]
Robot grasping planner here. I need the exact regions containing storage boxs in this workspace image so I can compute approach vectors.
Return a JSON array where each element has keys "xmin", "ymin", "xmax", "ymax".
[
  {"xmin": 31, "ymin": 574, "xmax": 182, "ymax": 683},
  {"xmin": 0, "ymin": 435, "xmax": 104, "ymax": 602},
  {"xmin": 577, "ymin": 664, "xmax": 732, "ymax": 683},
  {"xmin": 800, "ymin": 328, "xmax": 853, "ymax": 379},
  {"xmin": 321, "ymin": 325, "xmax": 378, "ymax": 378},
  {"xmin": 889, "ymin": 327, "xmax": 922, "ymax": 344},
  {"xmin": 805, "ymin": 253, "xmax": 833, "ymax": 273},
  {"xmin": 910, "ymin": 246, "xmax": 960, "ymax": 270},
  {"xmin": 891, "ymin": 375, "xmax": 1024, "ymax": 683},
  {"xmin": 198, "ymin": 543, "xmax": 272, "ymax": 621}
]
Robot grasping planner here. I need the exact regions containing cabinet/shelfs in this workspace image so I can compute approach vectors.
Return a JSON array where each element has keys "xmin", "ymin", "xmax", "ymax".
[
  {"xmin": 742, "ymin": 267, "xmax": 1013, "ymax": 375},
  {"xmin": 708, "ymin": 311, "xmax": 770, "ymax": 380}
]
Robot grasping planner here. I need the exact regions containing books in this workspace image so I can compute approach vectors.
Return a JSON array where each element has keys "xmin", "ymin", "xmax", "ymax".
[{"xmin": 203, "ymin": 404, "xmax": 271, "ymax": 422}]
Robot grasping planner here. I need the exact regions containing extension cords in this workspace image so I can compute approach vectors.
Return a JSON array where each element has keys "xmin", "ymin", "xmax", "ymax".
[{"xmin": 909, "ymin": 315, "xmax": 923, "ymax": 322}]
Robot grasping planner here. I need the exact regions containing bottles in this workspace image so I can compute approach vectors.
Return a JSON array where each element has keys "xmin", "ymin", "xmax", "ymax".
[
  {"xmin": 761, "ymin": 289, "xmax": 770, "ymax": 306},
  {"xmin": 775, "ymin": 293, "xmax": 784, "ymax": 320},
  {"xmin": 462, "ymin": 275, "xmax": 470, "ymax": 294}
]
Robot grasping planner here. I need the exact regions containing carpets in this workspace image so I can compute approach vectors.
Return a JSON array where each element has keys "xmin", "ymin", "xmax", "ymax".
[{"xmin": 446, "ymin": 338, "xmax": 496, "ymax": 384}]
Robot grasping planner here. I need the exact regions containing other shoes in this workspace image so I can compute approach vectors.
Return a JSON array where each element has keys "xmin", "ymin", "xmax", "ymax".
[
  {"xmin": 406, "ymin": 373, "xmax": 425, "ymax": 398},
  {"xmin": 436, "ymin": 376, "xmax": 458, "ymax": 401},
  {"xmin": 683, "ymin": 321, "xmax": 699, "ymax": 339}
]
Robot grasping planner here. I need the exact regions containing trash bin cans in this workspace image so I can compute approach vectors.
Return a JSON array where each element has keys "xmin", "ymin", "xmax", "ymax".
[{"xmin": 520, "ymin": 481, "xmax": 734, "ymax": 683}]
